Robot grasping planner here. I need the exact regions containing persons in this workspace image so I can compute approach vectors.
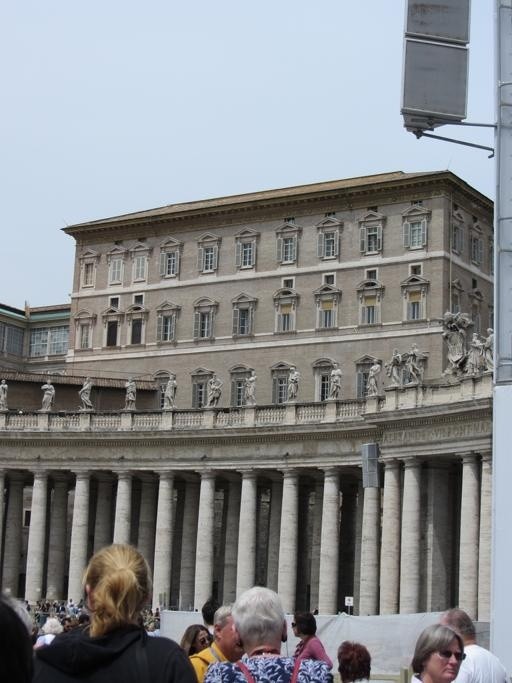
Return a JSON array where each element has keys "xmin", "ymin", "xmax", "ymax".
[
  {"xmin": 78, "ymin": 376, "xmax": 93, "ymax": 409},
  {"xmin": 243, "ymin": 370, "xmax": 258, "ymax": 404},
  {"xmin": 181, "ymin": 624, "xmax": 214, "ymax": 656},
  {"xmin": 0, "ymin": 379, "xmax": 10, "ymax": 411},
  {"xmin": 33, "ymin": 543, "xmax": 198, "ymax": 682},
  {"xmin": 202, "ymin": 600, "xmax": 220, "ymax": 635},
  {"xmin": 366, "ymin": 321, "xmax": 494, "ymax": 396},
  {"xmin": 202, "ymin": 586, "xmax": 332, "ymax": 683},
  {"xmin": 161, "ymin": 374, "xmax": 178, "ymax": 409},
  {"xmin": 328, "ymin": 363, "xmax": 342, "ymax": 398},
  {"xmin": 337, "ymin": 641, "xmax": 371, "ymax": 683},
  {"xmin": 286, "ymin": 366, "xmax": 300, "ymax": 401},
  {"xmin": 409, "ymin": 623, "xmax": 465, "ymax": 683},
  {"xmin": 41, "ymin": 379, "xmax": 57, "ymax": 411},
  {"xmin": 1, "ymin": 596, "xmax": 161, "ymax": 683},
  {"xmin": 188, "ymin": 603, "xmax": 246, "ymax": 683},
  {"xmin": 123, "ymin": 376, "xmax": 137, "ymax": 409},
  {"xmin": 204, "ymin": 373, "xmax": 223, "ymax": 406},
  {"xmin": 438, "ymin": 607, "xmax": 511, "ymax": 683},
  {"xmin": 291, "ymin": 610, "xmax": 333, "ymax": 671}
]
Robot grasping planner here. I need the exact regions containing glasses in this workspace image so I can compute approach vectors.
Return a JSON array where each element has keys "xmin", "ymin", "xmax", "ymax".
[{"xmin": 435, "ymin": 648, "xmax": 465, "ymax": 661}]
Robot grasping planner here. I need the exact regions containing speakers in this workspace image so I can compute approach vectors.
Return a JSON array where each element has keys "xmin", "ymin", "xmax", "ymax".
[
  {"xmin": 399, "ymin": 36, "xmax": 469, "ymax": 133},
  {"xmin": 403, "ymin": 0, "xmax": 470, "ymax": 45}
]
